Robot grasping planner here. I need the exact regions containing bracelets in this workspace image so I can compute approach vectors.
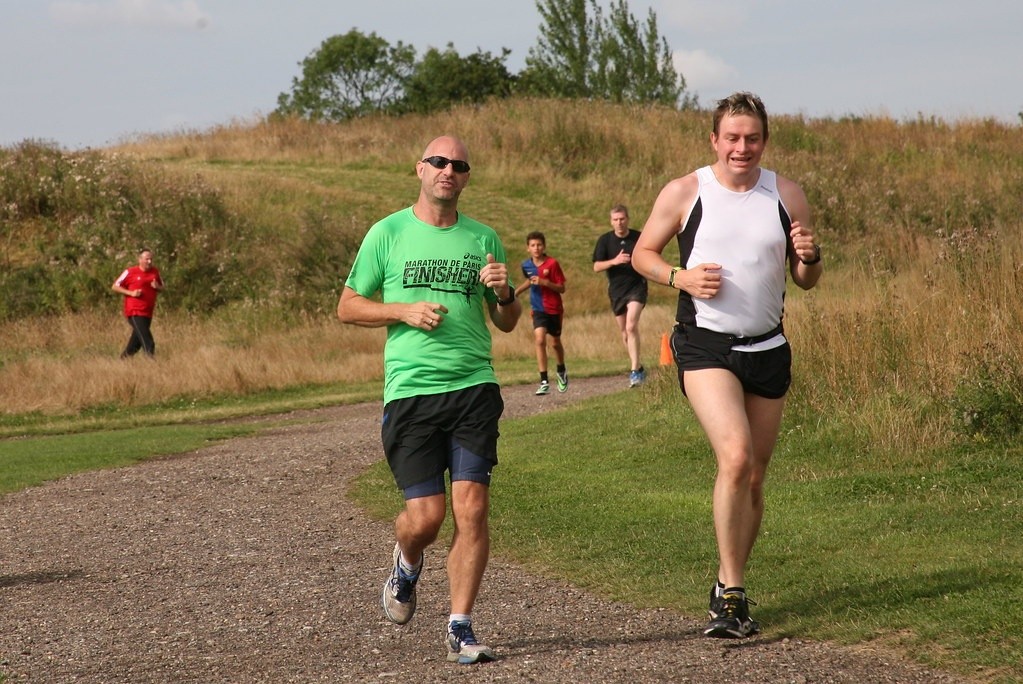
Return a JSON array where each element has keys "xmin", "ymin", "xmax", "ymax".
[{"xmin": 670, "ymin": 265, "xmax": 684, "ymax": 288}]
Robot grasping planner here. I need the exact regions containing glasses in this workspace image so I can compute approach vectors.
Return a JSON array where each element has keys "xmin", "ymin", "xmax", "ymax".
[{"xmin": 422, "ymin": 155, "xmax": 471, "ymax": 173}]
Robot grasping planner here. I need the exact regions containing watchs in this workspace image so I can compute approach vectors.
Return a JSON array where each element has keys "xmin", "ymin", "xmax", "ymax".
[
  {"xmin": 800, "ymin": 242, "xmax": 824, "ymax": 266},
  {"xmin": 495, "ymin": 285, "xmax": 516, "ymax": 305}
]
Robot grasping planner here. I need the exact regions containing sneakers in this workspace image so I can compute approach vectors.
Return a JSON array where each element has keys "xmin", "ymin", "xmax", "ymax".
[
  {"xmin": 536, "ymin": 384, "xmax": 551, "ymax": 395},
  {"xmin": 383, "ymin": 541, "xmax": 424, "ymax": 625},
  {"xmin": 446, "ymin": 621, "xmax": 495, "ymax": 664},
  {"xmin": 709, "ymin": 587, "xmax": 756, "ymax": 636},
  {"xmin": 630, "ymin": 369, "xmax": 645, "ymax": 387},
  {"xmin": 557, "ymin": 369, "xmax": 568, "ymax": 392},
  {"xmin": 709, "ymin": 597, "xmax": 756, "ymax": 638}
]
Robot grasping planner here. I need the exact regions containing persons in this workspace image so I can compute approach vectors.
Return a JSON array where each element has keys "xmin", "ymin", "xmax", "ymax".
[
  {"xmin": 110, "ymin": 249, "xmax": 166, "ymax": 363},
  {"xmin": 632, "ymin": 93, "xmax": 826, "ymax": 637},
  {"xmin": 593, "ymin": 202, "xmax": 650, "ymax": 388},
  {"xmin": 513, "ymin": 230, "xmax": 568, "ymax": 395},
  {"xmin": 338, "ymin": 132, "xmax": 526, "ymax": 665}
]
